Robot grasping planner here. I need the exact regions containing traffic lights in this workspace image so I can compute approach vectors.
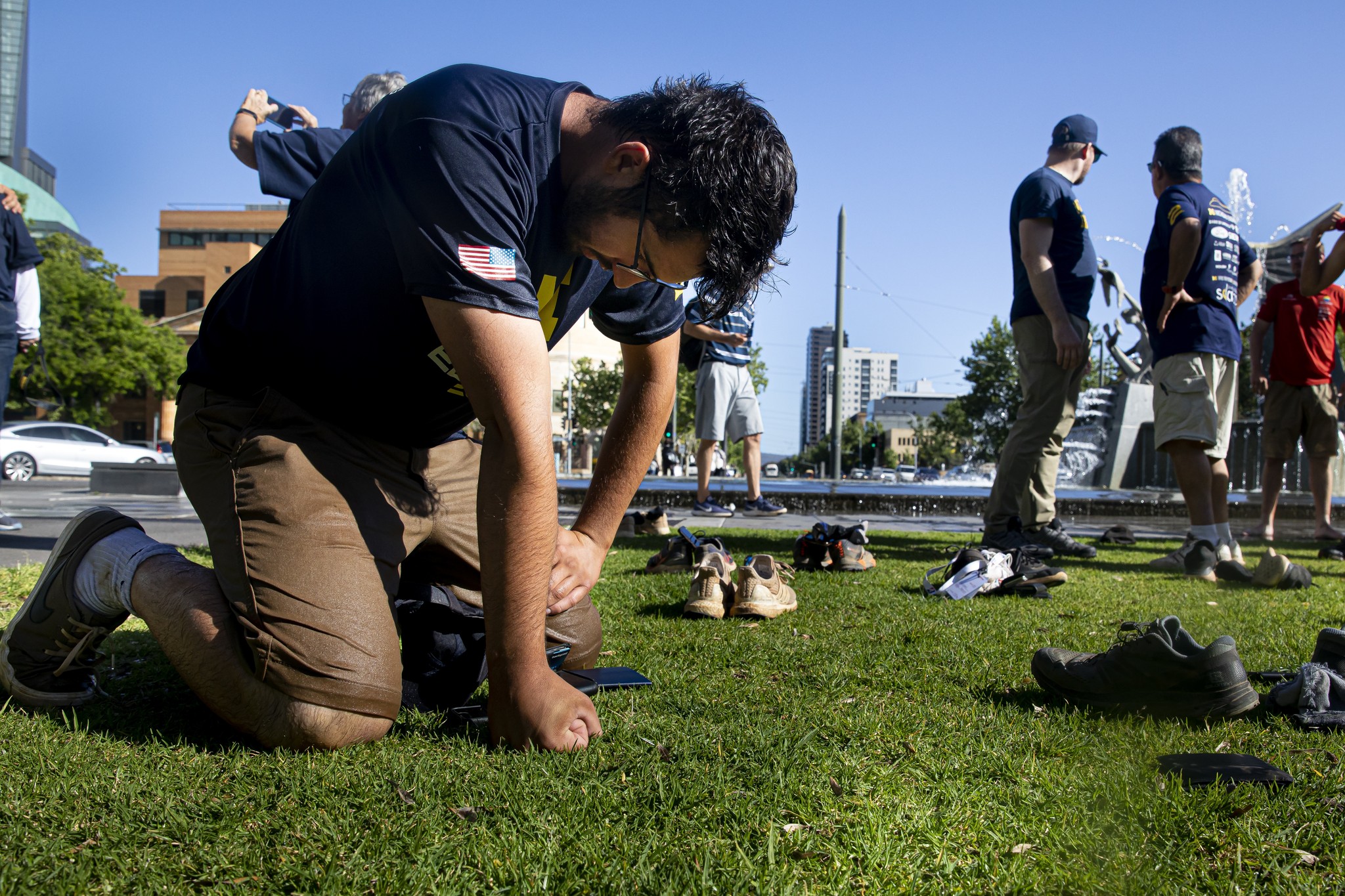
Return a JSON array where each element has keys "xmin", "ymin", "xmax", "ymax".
[{"xmin": 664, "ymin": 424, "xmax": 672, "ymax": 438}]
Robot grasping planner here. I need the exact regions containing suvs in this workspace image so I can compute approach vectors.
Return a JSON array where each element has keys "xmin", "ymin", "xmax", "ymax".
[{"xmin": 121, "ymin": 439, "xmax": 177, "ymax": 464}]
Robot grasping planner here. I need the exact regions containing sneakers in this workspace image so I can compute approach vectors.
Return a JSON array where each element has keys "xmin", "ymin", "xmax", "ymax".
[
  {"xmin": 742, "ymin": 494, "xmax": 788, "ymax": 516},
  {"xmin": 631, "ymin": 507, "xmax": 672, "ymax": 535},
  {"xmin": 0, "ymin": 506, "xmax": 146, "ymax": 707},
  {"xmin": 614, "ymin": 516, "xmax": 635, "ymax": 540},
  {"xmin": 690, "ymin": 496, "xmax": 734, "ymax": 517},
  {"xmin": 690, "ymin": 537, "xmax": 737, "ymax": 573},
  {"xmin": 1023, "ymin": 517, "xmax": 1096, "ymax": 558},
  {"xmin": 1150, "ymin": 539, "xmax": 1232, "ymax": 573},
  {"xmin": 940, "ymin": 541, "xmax": 986, "ymax": 582},
  {"xmin": 980, "ymin": 524, "xmax": 1054, "ymax": 560},
  {"xmin": 1318, "ymin": 541, "xmax": 1344, "ymax": 558},
  {"xmin": 645, "ymin": 529, "xmax": 707, "ymax": 573},
  {"xmin": 800, "ymin": 539, "xmax": 833, "ymax": 569},
  {"xmin": 729, "ymin": 554, "xmax": 798, "ymax": 618},
  {"xmin": 1183, "ymin": 539, "xmax": 1219, "ymax": 583},
  {"xmin": 986, "ymin": 544, "xmax": 1067, "ymax": 588},
  {"xmin": 1030, "ymin": 615, "xmax": 1260, "ymax": 724},
  {"xmin": 1250, "ymin": 546, "xmax": 1312, "ymax": 590},
  {"xmin": 0, "ymin": 510, "xmax": 23, "ymax": 530},
  {"xmin": 1308, "ymin": 627, "xmax": 1344, "ymax": 681},
  {"xmin": 834, "ymin": 539, "xmax": 876, "ymax": 571},
  {"xmin": 680, "ymin": 552, "xmax": 738, "ymax": 620}
]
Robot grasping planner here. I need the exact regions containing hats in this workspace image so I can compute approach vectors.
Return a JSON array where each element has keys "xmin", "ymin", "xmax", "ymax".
[
  {"xmin": 1100, "ymin": 527, "xmax": 1136, "ymax": 544},
  {"xmin": 1050, "ymin": 114, "xmax": 1108, "ymax": 157}
]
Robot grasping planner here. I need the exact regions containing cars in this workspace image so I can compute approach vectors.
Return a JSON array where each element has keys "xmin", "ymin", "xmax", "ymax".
[
  {"xmin": 0, "ymin": 420, "xmax": 167, "ymax": 482},
  {"xmin": 646, "ymin": 442, "xmax": 999, "ymax": 481}
]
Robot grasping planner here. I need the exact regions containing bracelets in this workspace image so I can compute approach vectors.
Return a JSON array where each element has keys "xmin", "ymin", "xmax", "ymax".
[{"xmin": 235, "ymin": 109, "xmax": 258, "ymax": 122}]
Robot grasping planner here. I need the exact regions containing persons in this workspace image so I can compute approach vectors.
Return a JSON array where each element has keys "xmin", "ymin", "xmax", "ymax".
[
  {"xmin": 680, "ymin": 260, "xmax": 787, "ymax": 517},
  {"xmin": 977, "ymin": 111, "xmax": 1110, "ymax": 558},
  {"xmin": 229, "ymin": 67, "xmax": 414, "ymax": 215},
  {"xmin": 0, "ymin": 183, "xmax": 24, "ymax": 216},
  {"xmin": 1, "ymin": 191, "xmax": 43, "ymax": 424},
  {"xmin": 1247, "ymin": 235, "xmax": 1344, "ymax": 541},
  {"xmin": 1139, "ymin": 124, "xmax": 1267, "ymax": 580},
  {"xmin": 0, "ymin": 61, "xmax": 798, "ymax": 753},
  {"xmin": 1297, "ymin": 211, "xmax": 1345, "ymax": 295},
  {"xmin": 653, "ymin": 432, "xmax": 730, "ymax": 477}
]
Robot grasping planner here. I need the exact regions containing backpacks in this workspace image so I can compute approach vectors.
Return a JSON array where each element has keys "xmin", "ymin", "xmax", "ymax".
[{"xmin": 391, "ymin": 582, "xmax": 488, "ymax": 711}]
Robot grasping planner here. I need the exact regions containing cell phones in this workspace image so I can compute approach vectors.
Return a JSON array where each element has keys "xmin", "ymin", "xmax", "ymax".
[{"xmin": 264, "ymin": 96, "xmax": 295, "ymax": 132}]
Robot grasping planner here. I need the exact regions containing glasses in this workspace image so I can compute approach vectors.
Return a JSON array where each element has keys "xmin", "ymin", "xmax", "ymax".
[
  {"xmin": 342, "ymin": 92, "xmax": 356, "ymax": 105},
  {"xmin": 1092, "ymin": 152, "xmax": 1102, "ymax": 163},
  {"xmin": 1147, "ymin": 162, "xmax": 1155, "ymax": 173},
  {"xmin": 616, "ymin": 135, "xmax": 689, "ymax": 291}
]
